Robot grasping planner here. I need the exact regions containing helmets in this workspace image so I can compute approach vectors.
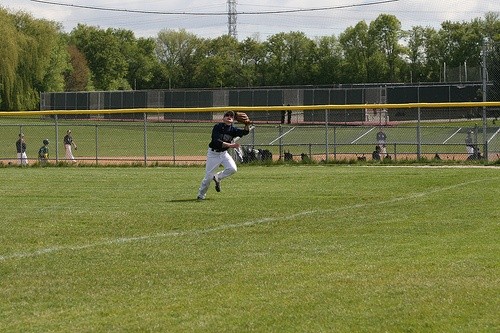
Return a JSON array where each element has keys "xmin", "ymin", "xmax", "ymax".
[{"xmin": 43, "ymin": 139, "xmax": 50, "ymax": 145}]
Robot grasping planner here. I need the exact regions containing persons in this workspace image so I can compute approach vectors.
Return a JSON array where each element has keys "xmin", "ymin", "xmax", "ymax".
[
  {"xmin": 282, "ymin": 104, "xmax": 292, "ymax": 123},
  {"xmin": 64, "ymin": 129, "xmax": 77, "ymax": 163},
  {"xmin": 466, "ymin": 131, "xmax": 473, "ymax": 155},
  {"xmin": 197, "ymin": 111, "xmax": 250, "ymax": 200},
  {"xmin": 373, "ymin": 146, "xmax": 380, "ymax": 160},
  {"xmin": 16, "ymin": 134, "xmax": 27, "ymax": 164},
  {"xmin": 39, "ymin": 139, "xmax": 50, "ymax": 163}
]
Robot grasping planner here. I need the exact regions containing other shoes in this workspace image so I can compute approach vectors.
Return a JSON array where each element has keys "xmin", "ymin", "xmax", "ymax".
[
  {"xmin": 212, "ymin": 176, "xmax": 220, "ymax": 192},
  {"xmin": 197, "ymin": 196, "xmax": 202, "ymax": 202}
]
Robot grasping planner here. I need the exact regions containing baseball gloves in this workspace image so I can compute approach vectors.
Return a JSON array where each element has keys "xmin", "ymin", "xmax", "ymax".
[{"xmin": 236, "ymin": 112, "xmax": 250, "ymax": 124}]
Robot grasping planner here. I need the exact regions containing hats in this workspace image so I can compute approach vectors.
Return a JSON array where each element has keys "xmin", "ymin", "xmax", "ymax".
[{"xmin": 224, "ymin": 110, "xmax": 234, "ymax": 117}]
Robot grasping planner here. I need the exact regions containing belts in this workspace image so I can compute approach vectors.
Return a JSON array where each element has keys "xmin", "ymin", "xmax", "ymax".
[{"xmin": 211, "ymin": 149, "xmax": 222, "ymax": 153}]
